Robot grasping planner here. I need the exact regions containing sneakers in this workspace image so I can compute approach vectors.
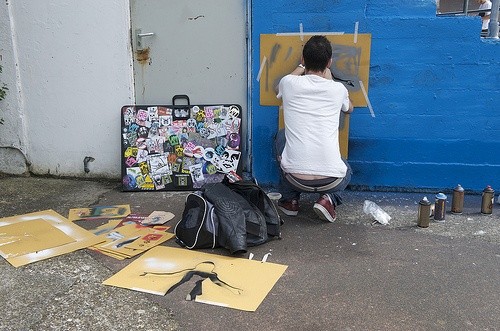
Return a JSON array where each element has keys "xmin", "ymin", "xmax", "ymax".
[
  {"xmin": 313, "ymin": 196, "xmax": 337, "ymax": 222},
  {"xmin": 277, "ymin": 199, "xmax": 299, "ymax": 216}
]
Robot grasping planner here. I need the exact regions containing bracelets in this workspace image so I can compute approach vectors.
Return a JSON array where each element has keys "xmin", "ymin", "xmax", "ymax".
[{"xmin": 299, "ymin": 63, "xmax": 306, "ymax": 70}]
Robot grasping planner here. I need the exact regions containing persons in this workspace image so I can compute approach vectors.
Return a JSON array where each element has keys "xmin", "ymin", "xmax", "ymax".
[
  {"xmin": 274, "ymin": 34, "xmax": 352, "ymax": 223},
  {"xmin": 476, "ymin": 0, "xmax": 493, "ymax": 38}
]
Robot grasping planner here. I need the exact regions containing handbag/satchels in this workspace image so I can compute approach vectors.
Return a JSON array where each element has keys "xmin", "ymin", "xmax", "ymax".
[{"xmin": 173, "ymin": 179, "xmax": 284, "ymax": 249}]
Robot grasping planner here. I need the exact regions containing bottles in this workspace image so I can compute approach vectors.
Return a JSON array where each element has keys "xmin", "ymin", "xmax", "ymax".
[
  {"xmin": 418, "ymin": 196, "xmax": 431, "ymax": 228},
  {"xmin": 451, "ymin": 184, "xmax": 465, "ymax": 214},
  {"xmin": 434, "ymin": 193, "xmax": 447, "ymax": 221},
  {"xmin": 481, "ymin": 185, "xmax": 494, "ymax": 214}
]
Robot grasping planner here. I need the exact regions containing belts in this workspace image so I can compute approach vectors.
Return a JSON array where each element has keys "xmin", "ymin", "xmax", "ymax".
[{"xmin": 282, "ymin": 169, "xmax": 343, "ymax": 192}]
